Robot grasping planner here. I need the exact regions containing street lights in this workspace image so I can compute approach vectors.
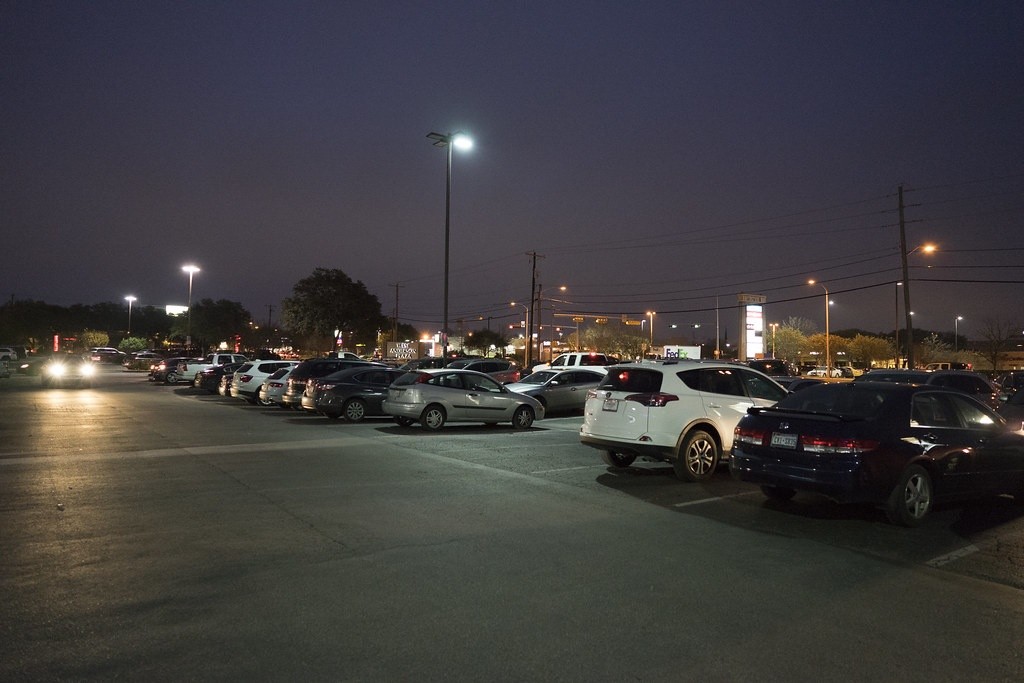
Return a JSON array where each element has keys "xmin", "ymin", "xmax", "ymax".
[
  {"xmin": 538, "ymin": 283, "xmax": 567, "ymax": 361},
  {"xmin": 894, "ymin": 281, "xmax": 903, "ymax": 370},
  {"xmin": 510, "ymin": 302, "xmax": 529, "ymax": 369},
  {"xmin": 124, "ymin": 295, "xmax": 137, "ymax": 348},
  {"xmin": 769, "ymin": 322, "xmax": 779, "ymax": 360},
  {"xmin": 181, "ymin": 263, "xmax": 200, "ymax": 357},
  {"xmin": 646, "ymin": 311, "xmax": 657, "ymax": 346},
  {"xmin": 954, "ymin": 315, "xmax": 963, "ymax": 353},
  {"xmin": 901, "ymin": 242, "xmax": 937, "ymax": 370},
  {"xmin": 425, "ymin": 129, "xmax": 474, "ymax": 386},
  {"xmin": 808, "ymin": 279, "xmax": 830, "ymax": 378}
]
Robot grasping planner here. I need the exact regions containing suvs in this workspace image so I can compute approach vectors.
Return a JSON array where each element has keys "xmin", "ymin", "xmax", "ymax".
[
  {"xmin": 89, "ymin": 346, "xmax": 127, "ymax": 363},
  {"xmin": 531, "ymin": 351, "xmax": 628, "ymax": 383},
  {"xmin": 428, "ymin": 357, "xmax": 521, "ymax": 392},
  {"xmin": 397, "ymin": 355, "xmax": 457, "ymax": 381}
]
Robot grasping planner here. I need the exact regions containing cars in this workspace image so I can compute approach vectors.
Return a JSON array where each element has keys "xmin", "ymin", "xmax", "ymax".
[
  {"xmin": 718, "ymin": 356, "xmax": 1024, "ymax": 425},
  {"xmin": 121, "ymin": 349, "xmax": 411, "ymax": 424},
  {"xmin": 0, "ymin": 344, "xmax": 45, "ymax": 379},
  {"xmin": 577, "ymin": 359, "xmax": 798, "ymax": 485},
  {"xmin": 500, "ymin": 368, "xmax": 609, "ymax": 421},
  {"xmin": 726, "ymin": 380, "xmax": 1024, "ymax": 530},
  {"xmin": 380, "ymin": 368, "xmax": 546, "ymax": 433},
  {"xmin": 40, "ymin": 354, "xmax": 92, "ymax": 389}
]
